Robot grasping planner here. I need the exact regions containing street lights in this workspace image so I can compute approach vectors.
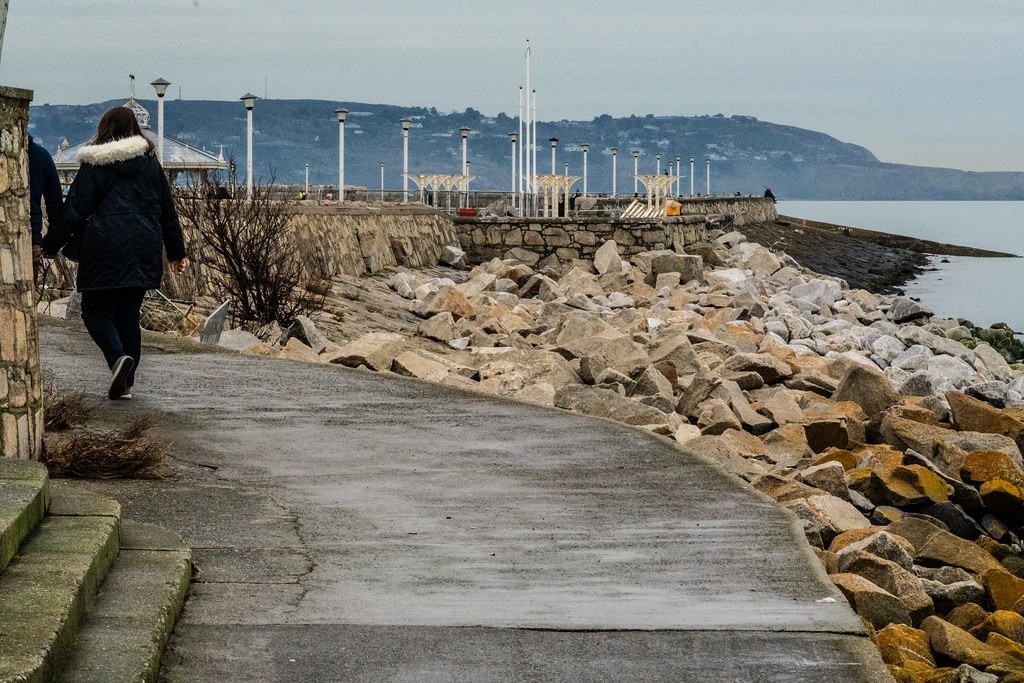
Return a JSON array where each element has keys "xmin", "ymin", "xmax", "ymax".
[
  {"xmin": 333, "ymin": 107, "xmax": 349, "ymax": 200},
  {"xmin": 549, "ymin": 138, "xmax": 560, "ymax": 217},
  {"xmin": 632, "ymin": 150, "xmax": 640, "ymax": 192},
  {"xmin": 610, "ymin": 147, "xmax": 619, "ymax": 197},
  {"xmin": 305, "ymin": 161, "xmax": 309, "ymax": 193},
  {"xmin": 655, "ymin": 153, "xmax": 663, "ymax": 176},
  {"xmin": 150, "ymin": 77, "xmax": 172, "ymax": 169},
  {"xmin": 705, "ymin": 157, "xmax": 711, "ymax": 196},
  {"xmin": 239, "ymin": 92, "xmax": 258, "ymax": 200},
  {"xmin": 688, "ymin": 155, "xmax": 696, "ymax": 195},
  {"xmin": 399, "ymin": 116, "xmax": 413, "ymax": 202},
  {"xmin": 580, "ymin": 143, "xmax": 590, "ymax": 197},
  {"xmin": 507, "ymin": 131, "xmax": 519, "ymax": 208},
  {"xmin": 379, "ymin": 161, "xmax": 385, "ymax": 201},
  {"xmin": 459, "ymin": 125, "xmax": 471, "ymax": 191},
  {"xmin": 675, "ymin": 153, "xmax": 683, "ymax": 196},
  {"xmin": 668, "ymin": 160, "xmax": 674, "ymax": 196}
]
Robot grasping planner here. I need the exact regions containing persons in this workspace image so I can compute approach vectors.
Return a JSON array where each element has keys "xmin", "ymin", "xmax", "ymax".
[
  {"xmin": 42, "ymin": 106, "xmax": 186, "ymax": 399},
  {"xmin": 215, "ymin": 187, "xmax": 232, "ymax": 199},
  {"xmin": 326, "ymin": 191, "xmax": 333, "ymax": 200},
  {"xmin": 558, "ymin": 196, "xmax": 564, "ymax": 217},
  {"xmin": 300, "ymin": 190, "xmax": 306, "ymax": 200},
  {"xmin": 764, "ymin": 185, "xmax": 777, "ymax": 204},
  {"xmin": 26, "ymin": 133, "xmax": 64, "ymax": 286},
  {"xmin": 569, "ymin": 196, "xmax": 574, "ymax": 211},
  {"xmin": 351, "ymin": 193, "xmax": 355, "ymax": 201}
]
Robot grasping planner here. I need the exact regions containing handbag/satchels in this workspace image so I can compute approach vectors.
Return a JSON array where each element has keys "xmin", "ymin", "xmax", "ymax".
[{"xmin": 62, "ymin": 213, "xmax": 91, "ymax": 263}]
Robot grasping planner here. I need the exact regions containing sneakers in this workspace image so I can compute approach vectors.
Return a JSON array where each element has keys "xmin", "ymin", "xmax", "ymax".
[{"xmin": 107, "ymin": 355, "xmax": 133, "ymax": 398}]
